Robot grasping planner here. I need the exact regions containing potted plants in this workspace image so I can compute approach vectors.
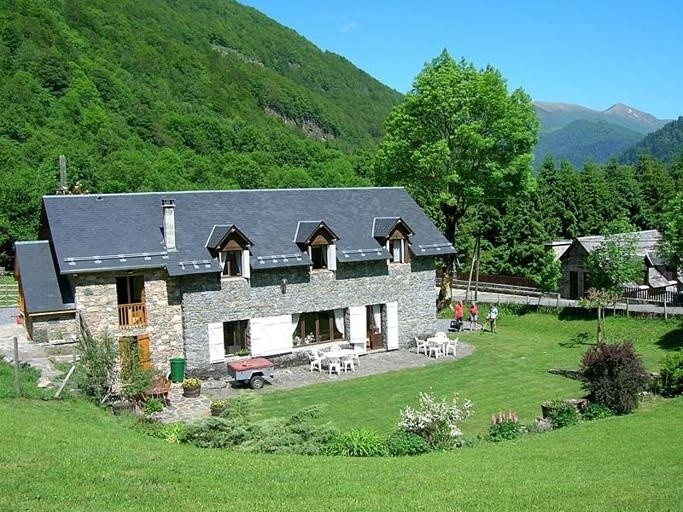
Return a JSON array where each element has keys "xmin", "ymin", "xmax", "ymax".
[{"xmin": 181, "ymin": 378, "xmax": 229, "ymax": 417}]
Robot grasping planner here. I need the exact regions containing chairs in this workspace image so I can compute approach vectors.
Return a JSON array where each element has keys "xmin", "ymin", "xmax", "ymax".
[
  {"xmin": 413, "ymin": 331, "xmax": 458, "ymax": 359},
  {"xmin": 305, "ymin": 342, "xmax": 360, "ymax": 376}
]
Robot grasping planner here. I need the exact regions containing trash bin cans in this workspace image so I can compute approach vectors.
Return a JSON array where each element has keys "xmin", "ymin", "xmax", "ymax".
[
  {"xmin": 370, "ymin": 334, "xmax": 383, "ymax": 349},
  {"xmin": 171, "ymin": 358, "xmax": 185, "ymax": 383}
]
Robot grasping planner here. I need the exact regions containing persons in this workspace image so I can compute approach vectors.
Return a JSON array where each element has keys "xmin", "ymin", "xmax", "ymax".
[{"xmin": 450, "ymin": 300, "xmax": 499, "ymax": 332}]
